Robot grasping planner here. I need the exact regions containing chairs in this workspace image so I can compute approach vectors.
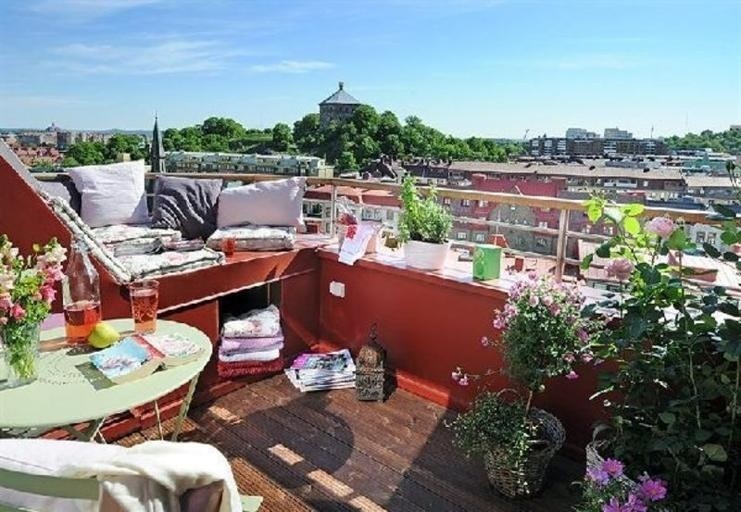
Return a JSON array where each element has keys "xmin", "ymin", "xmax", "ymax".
[{"xmin": 0, "ymin": 438, "xmax": 229, "ymax": 512}]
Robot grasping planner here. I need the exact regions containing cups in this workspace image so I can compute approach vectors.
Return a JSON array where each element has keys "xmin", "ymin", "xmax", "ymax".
[
  {"xmin": 514, "ymin": 256, "xmax": 537, "ymax": 273},
  {"xmin": 220, "ymin": 232, "xmax": 235, "ymax": 260},
  {"xmin": 471, "ymin": 242, "xmax": 501, "ymax": 279},
  {"xmin": 127, "ymin": 278, "xmax": 160, "ymax": 337}
]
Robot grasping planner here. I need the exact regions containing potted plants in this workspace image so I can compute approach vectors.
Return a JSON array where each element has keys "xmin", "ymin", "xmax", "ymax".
[{"xmin": 395, "ymin": 172, "xmax": 452, "ymax": 271}]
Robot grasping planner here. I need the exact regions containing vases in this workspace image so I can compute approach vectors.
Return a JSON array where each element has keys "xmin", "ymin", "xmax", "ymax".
[
  {"xmin": 478, "ymin": 410, "xmax": 567, "ymax": 498},
  {"xmin": 1, "ymin": 343, "xmax": 37, "ymax": 388},
  {"xmin": 586, "ymin": 442, "xmax": 674, "ymax": 512}
]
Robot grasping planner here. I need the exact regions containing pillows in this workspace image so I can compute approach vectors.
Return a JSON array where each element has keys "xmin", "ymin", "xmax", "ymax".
[
  {"xmin": 152, "ymin": 174, "xmax": 223, "ymax": 240},
  {"xmin": 216, "ymin": 175, "xmax": 309, "ymax": 232},
  {"xmin": 64, "ymin": 158, "xmax": 151, "ymax": 227}
]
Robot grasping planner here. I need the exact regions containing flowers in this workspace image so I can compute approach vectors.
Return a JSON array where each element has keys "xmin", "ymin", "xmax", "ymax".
[
  {"xmin": 579, "ymin": 458, "xmax": 667, "ymax": 510},
  {"xmin": 0, "ymin": 233, "xmax": 68, "ymax": 376},
  {"xmin": 448, "ymin": 270, "xmax": 602, "ymax": 454},
  {"xmin": 583, "ymin": 197, "xmax": 740, "ymax": 477}
]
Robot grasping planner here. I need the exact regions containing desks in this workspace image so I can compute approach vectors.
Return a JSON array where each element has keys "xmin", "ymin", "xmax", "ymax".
[{"xmin": 0, "ymin": 318, "xmax": 212, "ymax": 446}]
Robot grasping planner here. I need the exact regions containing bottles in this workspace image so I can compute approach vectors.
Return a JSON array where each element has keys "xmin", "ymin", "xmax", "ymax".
[{"xmin": 60, "ymin": 233, "xmax": 102, "ymax": 355}]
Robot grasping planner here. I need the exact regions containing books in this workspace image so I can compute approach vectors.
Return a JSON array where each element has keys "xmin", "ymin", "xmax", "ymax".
[
  {"xmin": 88, "ymin": 327, "xmax": 208, "ymax": 386},
  {"xmin": 283, "ymin": 347, "xmax": 357, "ymax": 393}
]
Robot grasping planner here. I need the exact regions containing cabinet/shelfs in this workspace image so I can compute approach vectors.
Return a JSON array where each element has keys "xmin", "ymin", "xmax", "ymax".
[{"xmin": 126, "ymin": 231, "xmax": 319, "ymax": 380}]
